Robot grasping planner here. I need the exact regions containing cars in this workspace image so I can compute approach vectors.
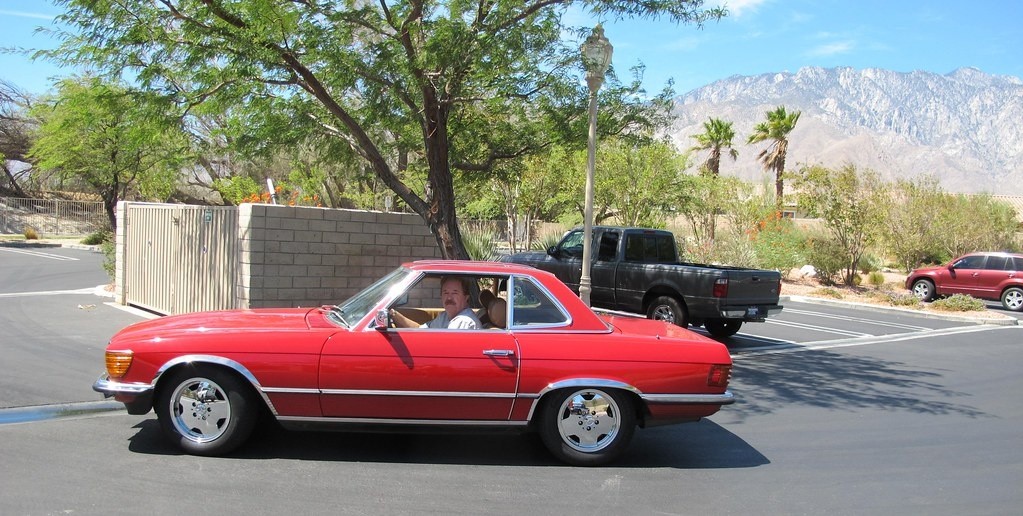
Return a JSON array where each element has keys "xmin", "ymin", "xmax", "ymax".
[
  {"xmin": 905, "ymin": 253, "xmax": 1023, "ymax": 313},
  {"xmin": 92, "ymin": 261, "xmax": 735, "ymax": 467}
]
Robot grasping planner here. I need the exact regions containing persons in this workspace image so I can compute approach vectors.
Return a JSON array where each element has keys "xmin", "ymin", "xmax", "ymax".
[{"xmin": 390, "ymin": 276, "xmax": 483, "ymax": 331}]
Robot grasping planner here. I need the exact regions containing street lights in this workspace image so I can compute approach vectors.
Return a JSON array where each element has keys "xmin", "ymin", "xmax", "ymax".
[{"xmin": 575, "ymin": 23, "xmax": 613, "ymax": 307}]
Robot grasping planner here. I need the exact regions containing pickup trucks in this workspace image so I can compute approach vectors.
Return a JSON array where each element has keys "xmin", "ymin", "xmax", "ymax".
[{"xmin": 494, "ymin": 225, "xmax": 783, "ymax": 338}]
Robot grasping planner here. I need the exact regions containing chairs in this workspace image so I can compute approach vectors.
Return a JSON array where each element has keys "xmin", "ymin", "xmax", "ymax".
[
  {"xmin": 475, "ymin": 289, "xmax": 496, "ymax": 325},
  {"xmin": 482, "ymin": 298, "xmax": 506, "ymax": 330}
]
